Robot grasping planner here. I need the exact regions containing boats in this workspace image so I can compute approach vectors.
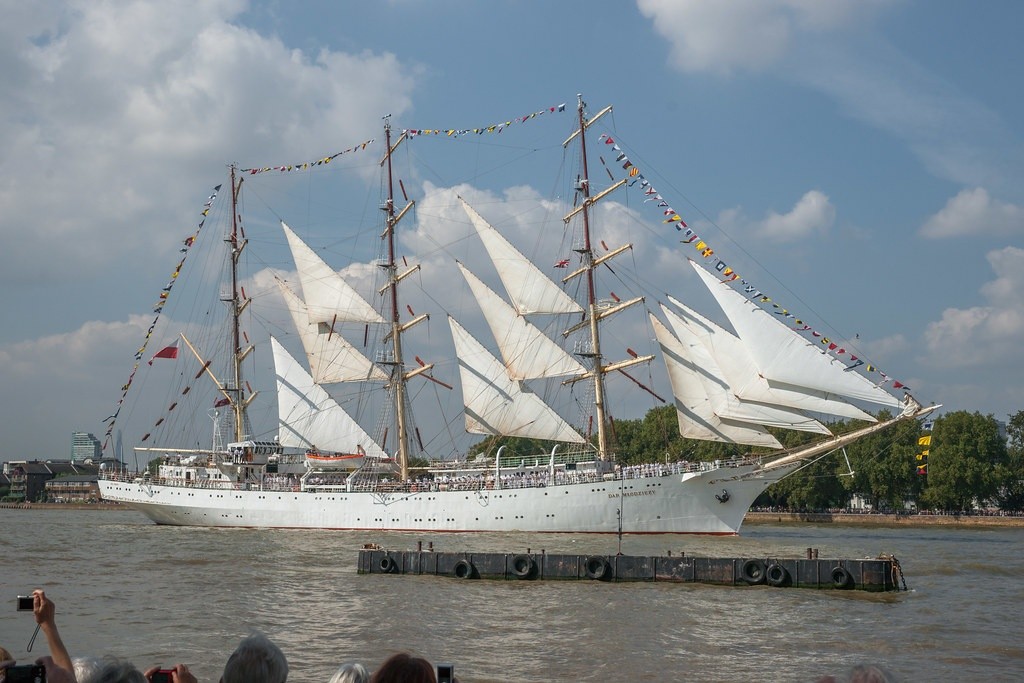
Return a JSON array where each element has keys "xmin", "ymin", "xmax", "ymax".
[{"xmin": 306, "ymin": 452, "xmax": 366, "ymax": 468}]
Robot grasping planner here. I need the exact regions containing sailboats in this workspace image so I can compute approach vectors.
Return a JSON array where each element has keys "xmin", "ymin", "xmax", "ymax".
[{"xmin": 96, "ymin": 93, "xmax": 944, "ymax": 537}]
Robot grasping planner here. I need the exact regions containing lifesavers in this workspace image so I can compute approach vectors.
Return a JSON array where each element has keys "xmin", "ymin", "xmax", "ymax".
[
  {"xmin": 830, "ymin": 567, "xmax": 850, "ymax": 588},
  {"xmin": 741, "ymin": 559, "xmax": 768, "ymax": 585},
  {"xmin": 452, "ymin": 560, "xmax": 473, "ymax": 580},
  {"xmin": 766, "ymin": 564, "xmax": 787, "ymax": 586},
  {"xmin": 379, "ymin": 557, "xmax": 393, "ymax": 573},
  {"xmin": 585, "ymin": 556, "xmax": 610, "ymax": 580},
  {"xmin": 508, "ymin": 554, "xmax": 534, "ymax": 577}
]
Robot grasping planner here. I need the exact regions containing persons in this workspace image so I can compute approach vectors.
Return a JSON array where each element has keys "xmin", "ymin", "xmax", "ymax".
[
  {"xmin": 178, "ymin": 452, "xmax": 181, "ymax": 461},
  {"xmin": 1, "ymin": 590, "xmax": 457, "ymax": 682},
  {"xmin": 407, "ymin": 469, "xmax": 593, "ymax": 491},
  {"xmin": 266, "ymin": 476, "xmax": 347, "ymax": 486},
  {"xmin": 163, "ymin": 452, "xmax": 170, "ymax": 464},
  {"xmin": 228, "ymin": 448, "xmax": 243, "ymax": 464},
  {"xmin": 614, "ymin": 459, "xmax": 689, "ymax": 480}
]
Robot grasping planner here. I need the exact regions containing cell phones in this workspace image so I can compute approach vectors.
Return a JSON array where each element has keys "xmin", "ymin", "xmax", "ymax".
[{"xmin": 436, "ymin": 663, "xmax": 454, "ymax": 683}]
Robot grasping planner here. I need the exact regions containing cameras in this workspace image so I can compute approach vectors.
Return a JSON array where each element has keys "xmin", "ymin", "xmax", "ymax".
[
  {"xmin": 4, "ymin": 664, "xmax": 46, "ymax": 683},
  {"xmin": 16, "ymin": 595, "xmax": 35, "ymax": 611},
  {"xmin": 151, "ymin": 669, "xmax": 178, "ymax": 683}
]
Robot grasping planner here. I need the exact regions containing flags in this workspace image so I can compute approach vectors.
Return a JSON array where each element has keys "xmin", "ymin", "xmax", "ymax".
[
  {"xmin": 400, "ymin": 102, "xmax": 566, "ymax": 139},
  {"xmin": 242, "ymin": 137, "xmax": 375, "ymax": 174},
  {"xmin": 916, "ymin": 421, "xmax": 934, "ymax": 475},
  {"xmin": 597, "ymin": 133, "xmax": 911, "ymax": 393},
  {"xmin": 99, "ymin": 183, "xmax": 221, "ymax": 457},
  {"xmin": 148, "ymin": 338, "xmax": 179, "ymax": 365}
]
